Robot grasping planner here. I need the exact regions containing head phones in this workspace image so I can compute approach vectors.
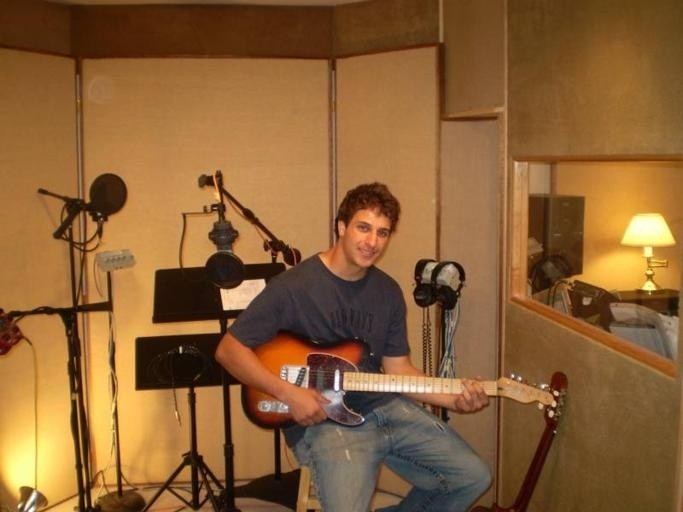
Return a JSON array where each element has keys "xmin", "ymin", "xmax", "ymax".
[
  {"xmin": 431, "ymin": 261, "xmax": 465, "ymax": 310},
  {"xmin": 414, "ymin": 258, "xmax": 436, "ymax": 307}
]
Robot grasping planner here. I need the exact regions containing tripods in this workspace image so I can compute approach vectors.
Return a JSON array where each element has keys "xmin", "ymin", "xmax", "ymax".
[{"xmin": 141, "ymin": 388, "xmax": 225, "ymax": 512}]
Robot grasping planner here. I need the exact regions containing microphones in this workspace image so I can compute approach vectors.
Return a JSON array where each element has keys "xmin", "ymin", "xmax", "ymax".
[
  {"xmin": 205, "ymin": 221, "xmax": 246, "ymax": 289},
  {"xmin": 89, "ymin": 173, "xmax": 127, "ymax": 216}
]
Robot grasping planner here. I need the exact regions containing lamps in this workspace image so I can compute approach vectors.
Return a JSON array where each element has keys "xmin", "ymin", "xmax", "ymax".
[{"xmin": 621, "ymin": 212, "xmax": 677, "ymax": 294}]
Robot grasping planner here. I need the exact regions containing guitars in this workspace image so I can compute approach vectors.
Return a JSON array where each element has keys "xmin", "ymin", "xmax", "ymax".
[
  {"xmin": 240, "ymin": 330, "xmax": 565, "ymax": 428},
  {"xmin": 475, "ymin": 371, "xmax": 568, "ymax": 511}
]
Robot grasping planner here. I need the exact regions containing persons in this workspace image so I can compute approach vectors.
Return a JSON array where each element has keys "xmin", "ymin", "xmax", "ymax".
[{"xmin": 210, "ymin": 179, "xmax": 494, "ymax": 511}]
